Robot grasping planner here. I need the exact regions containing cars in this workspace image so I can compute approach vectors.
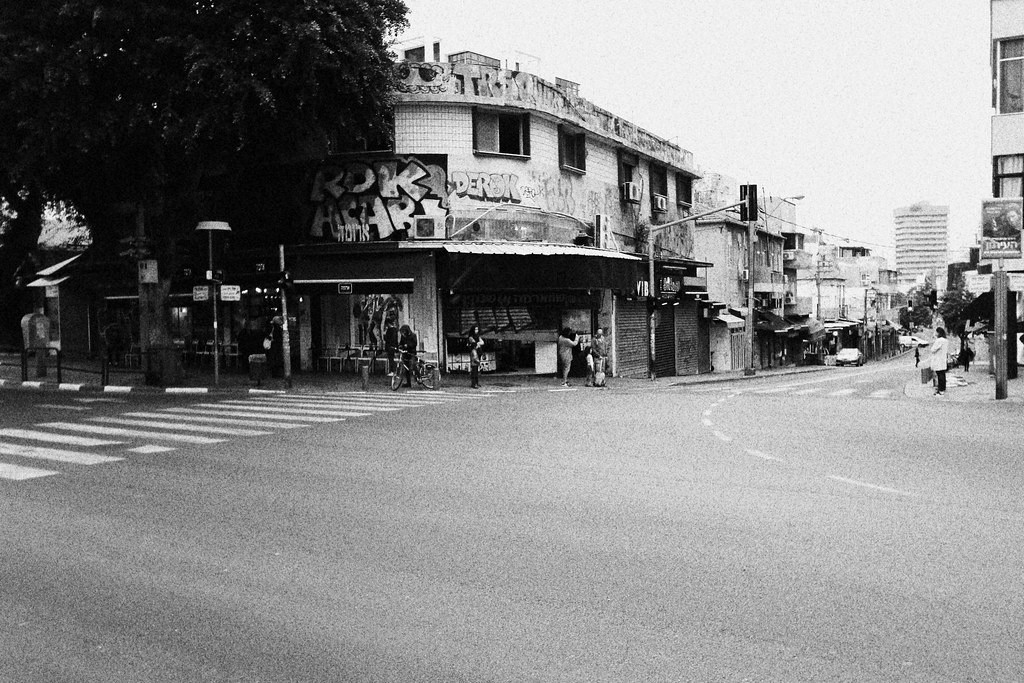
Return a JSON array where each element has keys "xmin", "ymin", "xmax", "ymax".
[{"xmin": 836, "ymin": 348, "xmax": 864, "ymax": 368}]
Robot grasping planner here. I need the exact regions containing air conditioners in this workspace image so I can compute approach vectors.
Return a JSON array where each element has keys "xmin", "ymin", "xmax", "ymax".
[
  {"xmin": 784, "ymin": 296, "xmax": 797, "ymax": 306},
  {"xmin": 413, "ymin": 215, "xmax": 446, "ymax": 240},
  {"xmin": 592, "ymin": 213, "xmax": 612, "ymax": 249},
  {"xmin": 782, "ymin": 251, "xmax": 795, "ymax": 261},
  {"xmin": 742, "ymin": 270, "xmax": 750, "ymax": 281},
  {"xmin": 654, "ymin": 196, "xmax": 666, "ymax": 210},
  {"xmin": 623, "ymin": 182, "xmax": 641, "ymax": 202}
]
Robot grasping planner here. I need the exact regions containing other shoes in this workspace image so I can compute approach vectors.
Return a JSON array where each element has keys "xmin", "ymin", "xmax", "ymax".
[
  {"xmin": 561, "ymin": 381, "xmax": 573, "ymax": 389},
  {"xmin": 387, "ymin": 372, "xmax": 396, "ymax": 377},
  {"xmin": 471, "ymin": 384, "xmax": 482, "ymax": 389},
  {"xmin": 934, "ymin": 391, "xmax": 945, "ymax": 396}
]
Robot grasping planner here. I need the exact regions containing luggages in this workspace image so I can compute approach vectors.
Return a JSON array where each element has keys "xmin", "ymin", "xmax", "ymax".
[{"xmin": 594, "ymin": 357, "xmax": 607, "ymax": 387}]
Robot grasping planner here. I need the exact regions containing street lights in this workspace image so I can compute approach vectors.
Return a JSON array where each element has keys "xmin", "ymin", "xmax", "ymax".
[{"xmin": 744, "ymin": 195, "xmax": 805, "ymax": 376}]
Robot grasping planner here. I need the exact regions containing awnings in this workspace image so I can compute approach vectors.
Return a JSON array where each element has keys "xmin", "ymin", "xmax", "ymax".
[
  {"xmin": 753, "ymin": 309, "xmax": 790, "ymax": 330},
  {"xmin": 27, "ymin": 253, "xmax": 85, "ymax": 288},
  {"xmin": 716, "ymin": 314, "xmax": 745, "ymax": 328},
  {"xmin": 288, "ymin": 251, "xmax": 418, "ymax": 293}
]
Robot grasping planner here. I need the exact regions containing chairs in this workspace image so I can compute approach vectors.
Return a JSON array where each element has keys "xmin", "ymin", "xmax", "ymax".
[
  {"xmin": 124, "ymin": 338, "xmax": 239, "ymax": 368},
  {"xmin": 316, "ymin": 345, "xmax": 401, "ymax": 375}
]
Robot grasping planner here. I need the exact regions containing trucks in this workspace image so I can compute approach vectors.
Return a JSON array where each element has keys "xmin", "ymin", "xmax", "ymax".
[{"xmin": 899, "ymin": 336, "xmax": 930, "ymax": 349}]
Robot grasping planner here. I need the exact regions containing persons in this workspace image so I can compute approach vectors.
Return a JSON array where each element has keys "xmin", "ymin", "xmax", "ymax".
[
  {"xmin": 990, "ymin": 202, "xmax": 1021, "ymax": 250},
  {"xmin": 583, "ymin": 346, "xmax": 593, "ymax": 387},
  {"xmin": 931, "ymin": 327, "xmax": 949, "ymax": 396},
  {"xmin": 591, "ymin": 328, "xmax": 608, "ymax": 371},
  {"xmin": 469, "ymin": 324, "xmax": 486, "ymax": 389},
  {"xmin": 557, "ymin": 326, "xmax": 579, "ymax": 385},
  {"xmin": 383, "ymin": 317, "xmax": 399, "ymax": 377},
  {"xmin": 914, "ymin": 348, "xmax": 920, "ymax": 368},
  {"xmin": 358, "ymin": 293, "xmax": 403, "ymax": 349},
  {"xmin": 470, "ymin": 342, "xmax": 480, "ymax": 388},
  {"xmin": 961, "ymin": 342, "xmax": 973, "ymax": 372},
  {"xmin": 396, "ymin": 325, "xmax": 423, "ymax": 388}
]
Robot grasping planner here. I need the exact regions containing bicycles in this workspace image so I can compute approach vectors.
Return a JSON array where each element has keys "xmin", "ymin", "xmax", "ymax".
[{"xmin": 389, "ymin": 345, "xmax": 442, "ymax": 391}]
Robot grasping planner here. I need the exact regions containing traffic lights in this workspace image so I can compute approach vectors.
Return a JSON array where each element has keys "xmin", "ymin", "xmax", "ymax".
[{"xmin": 907, "ymin": 300, "xmax": 913, "ymax": 312}]
